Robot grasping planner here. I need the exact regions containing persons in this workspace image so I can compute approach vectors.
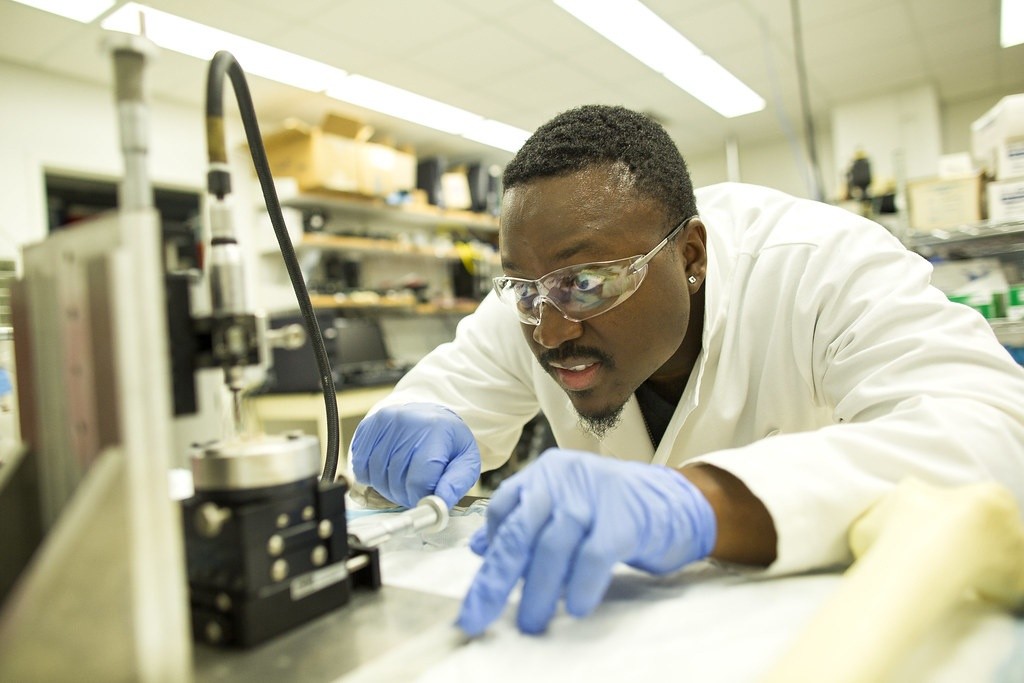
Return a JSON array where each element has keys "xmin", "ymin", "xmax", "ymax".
[{"xmin": 348, "ymin": 105, "xmax": 1024, "ymax": 635}]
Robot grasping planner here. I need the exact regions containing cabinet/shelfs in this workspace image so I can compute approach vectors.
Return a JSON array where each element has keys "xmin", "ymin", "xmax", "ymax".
[
  {"xmin": 900, "ymin": 221, "xmax": 1024, "ymax": 347},
  {"xmin": 251, "ymin": 189, "xmax": 501, "ymax": 322}
]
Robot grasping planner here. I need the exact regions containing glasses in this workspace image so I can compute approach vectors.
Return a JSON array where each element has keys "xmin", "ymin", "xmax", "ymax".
[{"xmin": 491, "ymin": 214, "xmax": 703, "ymax": 326}]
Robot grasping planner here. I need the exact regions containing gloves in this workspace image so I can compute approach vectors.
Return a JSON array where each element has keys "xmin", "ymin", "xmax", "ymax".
[
  {"xmin": 456, "ymin": 447, "xmax": 719, "ymax": 636},
  {"xmin": 350, "ymin": 403, "xmax": 481, "ymax": 512}
]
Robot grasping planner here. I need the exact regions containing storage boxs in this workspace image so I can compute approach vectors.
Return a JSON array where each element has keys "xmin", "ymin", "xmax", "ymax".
[
  {"xmin": 904, "ymin": 176, "xmax": 983, "ymax": 234},
  {"xmin": 970, "ymin": 93, "xmax": 1024, "ymax": 179},
  {"xmin": 980, "ymin": 179, "xmax": 1024, "ymax": 225},
  {"xmin": 236, "ymin": 113, "xmax": 418, "ymax": 200}
]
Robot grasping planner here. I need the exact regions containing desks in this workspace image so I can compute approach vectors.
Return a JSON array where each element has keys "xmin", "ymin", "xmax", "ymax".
[
  {"xmin": 238, "ymin": 386, "xmax": 393, "ymax": 473},
  {"xmin": 192, "ymin": 491, "xmax": 1024, "ymax": 683}
]
